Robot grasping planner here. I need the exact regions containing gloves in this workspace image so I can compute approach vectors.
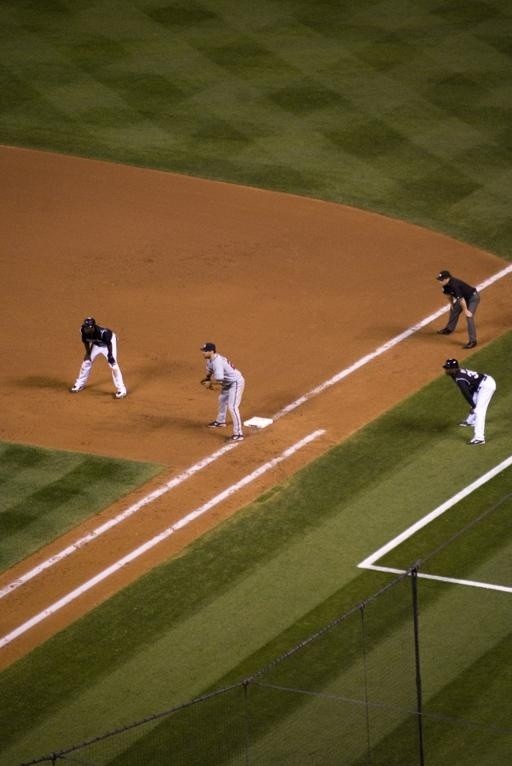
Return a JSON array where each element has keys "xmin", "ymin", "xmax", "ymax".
[{"xmin": 108, "ymin": 353, "xmax": 115, "ymax": 364}]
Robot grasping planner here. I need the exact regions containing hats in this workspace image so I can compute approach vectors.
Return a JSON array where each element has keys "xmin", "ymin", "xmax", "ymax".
[
  {"xmin": 436, "ymin": 271, "xmax": 449, "ymax": 279},
  {"xmin": 201, "ymin": 343, "xmax": 215, "ymax": 350}
]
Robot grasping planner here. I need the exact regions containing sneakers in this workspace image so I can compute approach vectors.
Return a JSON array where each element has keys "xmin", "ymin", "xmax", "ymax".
[
  {"xmin": 226, "ymin": 436, "xmax": 244, "ymax": 442},
  {"xmin": 467, "ymin": 438, "xmax": 485, "ymax": 444},
  {"xmin": 71, "ymin": 386, "xmax": 84, "ymax": 391},
  {"xmin": 465, "ymin": 341, "xmax": 476, "ymax": 348},
  {"xmin": 438, "ymin": 328, "xmax": 451, "ymax": 334},
  {"xmin": 207, "ymin": 421, "xmax": 226, "ymax": 428},
  {"xmin": 458, "ymin": 420, "xmax": 475, "ymax": 427},
  {"xmin": 115, "ymin": 392, "xmax": 127, "ymax": 398}
]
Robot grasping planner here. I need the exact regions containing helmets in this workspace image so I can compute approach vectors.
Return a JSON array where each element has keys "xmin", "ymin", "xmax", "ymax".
[
  {"xmin": 82, "ymin": 318, "xmax": 95, "ymax": 328},
  {"xmin": 443, "ymin": 359, "xmax": 458, "ymax": 368}
]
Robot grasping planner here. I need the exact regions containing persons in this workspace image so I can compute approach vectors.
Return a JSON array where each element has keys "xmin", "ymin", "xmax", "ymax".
[
  {"xmin": 69, "ymin": 317, "xmax": 126, "ymax": 397},
  {"xmin": 197, "ymin": 339, "xmax": 247, "ymax": 444},
  {"xmin": 435, "ymin": 266, "xmax": 481, "ymax": 349},
  {"xmin": 442, "ymin": 355, "xmax": 497, "ymax": 448}
]
